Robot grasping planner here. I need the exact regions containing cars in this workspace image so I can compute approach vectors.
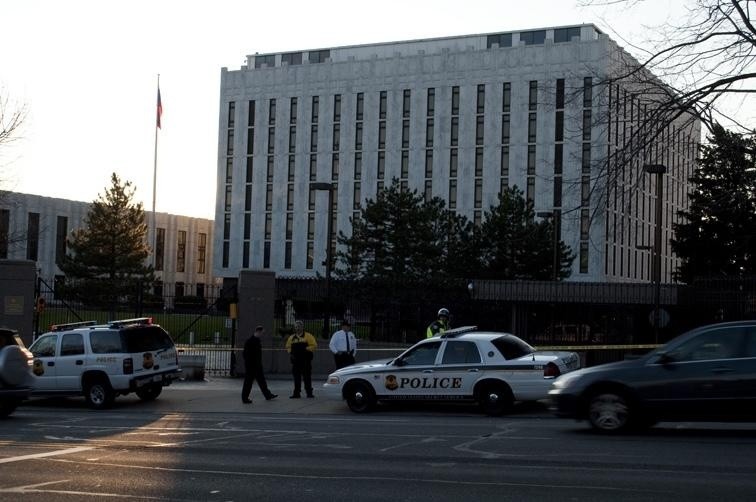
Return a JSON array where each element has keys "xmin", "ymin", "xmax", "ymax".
[
  {"xmin": 546, "ymin": 319, "xmax": 756, "ymax": 434},
  {"xmin": 322, "ymin": 324, "xmax": 582, "ymax": 413}
]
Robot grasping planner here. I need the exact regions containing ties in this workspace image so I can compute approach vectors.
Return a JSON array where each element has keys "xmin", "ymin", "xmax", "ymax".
[{"xmin": 346, "ymin": 333, "xmax": 350, "ymax": 353}]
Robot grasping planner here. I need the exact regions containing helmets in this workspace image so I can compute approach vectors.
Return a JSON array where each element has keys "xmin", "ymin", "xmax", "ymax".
[{"xmin": 438, "ymin": 308, "xmax": 450, "ymax": 317}]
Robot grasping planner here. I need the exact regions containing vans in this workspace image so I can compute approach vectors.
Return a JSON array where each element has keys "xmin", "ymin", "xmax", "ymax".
[
  {"xmin": 573, "ymin": 321, "xmax": 603, "ymax": 344},
  {"xmin": 528, "ymin": 322, "xmax": 590, "ymax": 342}
]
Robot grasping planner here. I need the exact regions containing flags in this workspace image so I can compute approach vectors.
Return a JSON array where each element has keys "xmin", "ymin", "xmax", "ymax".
[{"xmin": 157, "ymin": 88, "xmax": 162, "ymax": 127}]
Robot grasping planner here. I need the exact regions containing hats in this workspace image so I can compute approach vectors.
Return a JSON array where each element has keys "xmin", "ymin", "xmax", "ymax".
[{"xmin": 340, "ymin": 319, "xmax": 351, "ymax": 326}]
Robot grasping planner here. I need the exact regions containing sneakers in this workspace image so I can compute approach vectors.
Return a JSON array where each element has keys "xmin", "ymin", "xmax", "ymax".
[
  {"xmin": 243, "ymin": 398, "xmax": 252, "ymax": 404},
  {"xmin": 306, "ymin": 393, "xmax": 315, "ymax": 398},
  {"xmin": 265, "ymin": 394, "xmax": 279, "ymax": 401},
  {"xmin": 289, "ymin": 393, "xmax": 302, "ymax": 399}
]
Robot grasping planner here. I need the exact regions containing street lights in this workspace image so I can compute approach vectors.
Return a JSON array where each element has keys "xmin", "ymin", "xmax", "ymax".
[
  {"xmin": 310, "ymin": 181, "xmax": 334, "ymax": 340},
  {"xmin": 641, "ymin": 163, "xmax": 667, "ymax": 344}
]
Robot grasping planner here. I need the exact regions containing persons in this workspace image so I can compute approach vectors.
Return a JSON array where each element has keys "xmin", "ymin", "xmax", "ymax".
[
  {"xmin": 426, "ymin": 308, "xmax": 450, "ymax": 338},
  {"xmin": 241, "ymin": 327, "xmax": 278, "ymax": 403},
  {"xmin": 329, "ymin": 321, "xmax": 357, "ymax": 370},
  {"xmin": 285, "ymin": 319, "xmax": 318, "ymax": 398}
]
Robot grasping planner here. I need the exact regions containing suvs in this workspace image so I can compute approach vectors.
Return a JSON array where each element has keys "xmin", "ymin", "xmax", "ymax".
[
  {"xmin": 26, "ymin": 317, "xmax": 182, "ymax": 409},
  {"xmin": 0, "ymin": 328, "xmax": 34, "ymax": 419}
]
